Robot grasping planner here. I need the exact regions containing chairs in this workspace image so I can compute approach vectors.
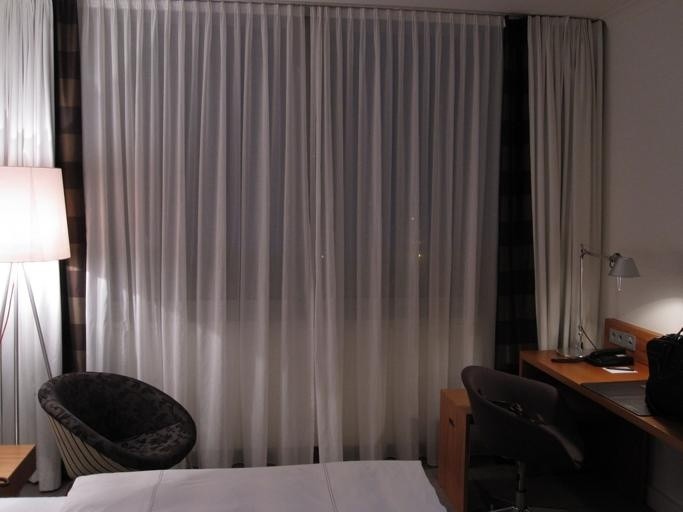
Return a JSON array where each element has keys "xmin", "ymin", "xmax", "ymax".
[
  {"xmin": 38, "ymin": 370, "xmax": 197, "ymax": 480},
  {"xmin": 459, "ymin": 365, "xmax": 573, "ymax": 512}
]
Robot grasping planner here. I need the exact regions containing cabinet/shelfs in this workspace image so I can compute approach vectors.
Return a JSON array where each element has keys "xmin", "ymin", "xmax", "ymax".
[{"xmin": 436, "ymin": 386, "xmax": 495, "ymax": 512}]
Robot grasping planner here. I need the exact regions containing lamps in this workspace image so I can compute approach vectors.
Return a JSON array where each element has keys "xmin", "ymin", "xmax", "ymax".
[
  {"xmin": 557, "ymin": 241, "xmax": 642, "ymax": 359},
  {"xmin": 0, "ymin": 166, "xmax": 73, "ymax": 441}
]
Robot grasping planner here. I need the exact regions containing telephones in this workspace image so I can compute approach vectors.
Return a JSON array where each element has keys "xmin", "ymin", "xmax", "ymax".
[{"xmin": 586, "ymin": 348, "xmax": 634, "ymax": 367}]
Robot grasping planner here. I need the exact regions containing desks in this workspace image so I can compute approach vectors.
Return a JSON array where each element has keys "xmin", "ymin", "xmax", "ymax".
[
  {"xmin": 517, "ymin": 316, "xmax": 683, "ymax": 469},
  {"xmin": 0, "ymin": 444, "xmax": 39, "ymax": 497}
]
